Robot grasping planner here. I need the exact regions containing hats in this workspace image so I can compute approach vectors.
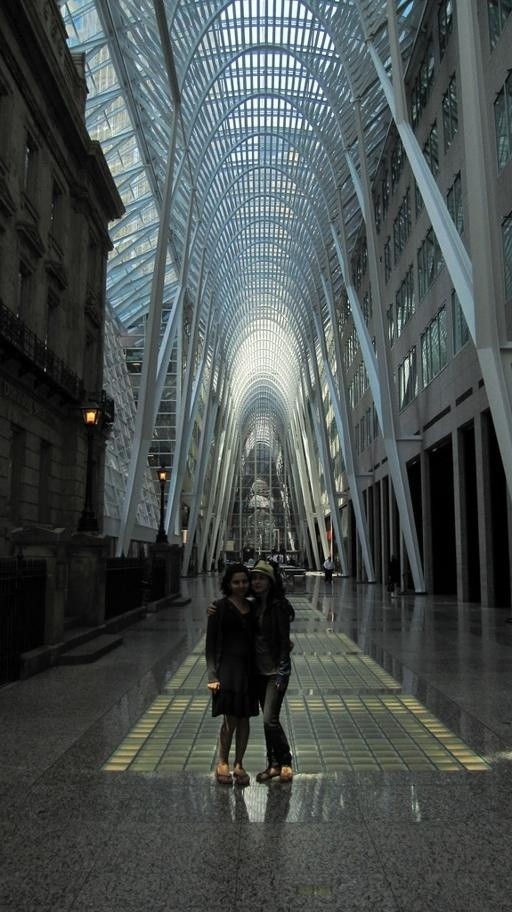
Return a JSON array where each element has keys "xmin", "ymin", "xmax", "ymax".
[{"xmin": 250, "ymin": 559, "xmax": 275, "ymax": 582}]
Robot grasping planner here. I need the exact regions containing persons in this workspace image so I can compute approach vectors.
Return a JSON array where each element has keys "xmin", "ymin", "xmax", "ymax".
[
  {"xmin": 207, "ymin": 558, "xmax": 297, "ymax": 783},
  {"xmin": 202, "ymin": 565, "xmax": 263, "ymax": 785},
  {"xmin": 386, "ymin": 554, "xmax": 401, "ymax": 598},
  {"xmin": 323, "ymin": 557, "xmax": 335, "ymax": 583}
]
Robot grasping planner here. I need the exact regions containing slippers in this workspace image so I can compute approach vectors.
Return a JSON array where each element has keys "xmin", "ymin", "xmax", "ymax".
[
  {"xmin": 215, "ymin": 769, "xmax": 233, "ymax": 783},
  {"xmin": 256, "ymin": 769, "xmax": 280, "ymax": 782},
  {"xmin": 234, "ymin": 772, "xmax": 250, "ymax": 785},
  {"xmin": 281, "ymin": 766, "xmax": 292, "ymax": 783}
]
